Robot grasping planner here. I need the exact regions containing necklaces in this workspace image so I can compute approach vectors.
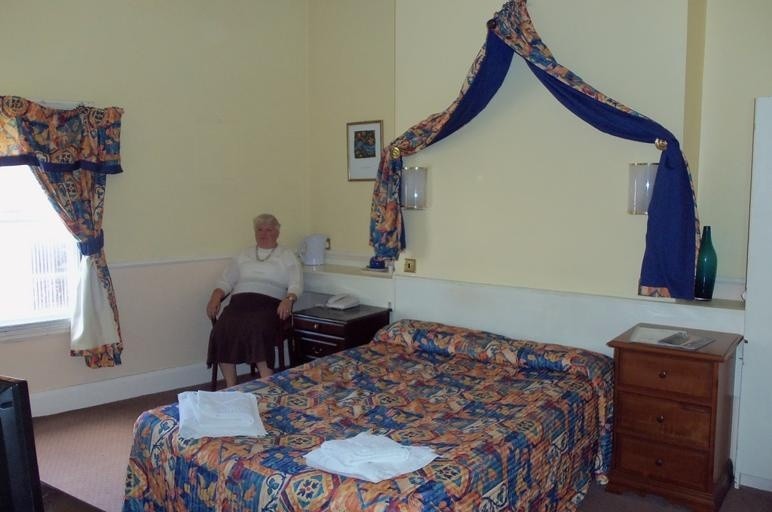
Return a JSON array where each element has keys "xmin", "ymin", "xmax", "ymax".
[{"xmin": 255, "ymin": 244, "xmax": 276, "ymax": 261}]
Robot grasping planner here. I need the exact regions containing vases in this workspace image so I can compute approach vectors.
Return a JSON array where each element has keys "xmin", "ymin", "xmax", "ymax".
[{"xmin": 694, "ymin": 226, "xmax": 717, "ymax": 302}]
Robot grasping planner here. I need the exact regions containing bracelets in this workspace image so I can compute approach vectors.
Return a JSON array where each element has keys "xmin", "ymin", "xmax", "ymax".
[{"xmin": 287, "ymin": 295, "xmax": 295, "ymax": 301}]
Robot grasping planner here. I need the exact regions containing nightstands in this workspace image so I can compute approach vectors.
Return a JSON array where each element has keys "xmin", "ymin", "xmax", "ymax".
[
  {"xmin": 292, "ymin": 305, "xmax": 391, "ymax": 366},
  {"xmin": 605, "ymin": 322, "xmax": 744, "ymax": 512}
]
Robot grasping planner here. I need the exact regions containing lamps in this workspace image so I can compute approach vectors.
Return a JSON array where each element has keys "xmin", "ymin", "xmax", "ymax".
[
  {"xmin": 628, "ymin": 162, "xmax": 659, "ymax": 217},
  {"xmin": 399, "ymin": 166, "xmax": 428, "ymax": 210}
]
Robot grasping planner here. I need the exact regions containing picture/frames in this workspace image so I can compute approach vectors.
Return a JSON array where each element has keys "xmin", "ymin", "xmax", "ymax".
[{"xmin": 346, "ymin": 120, "xmax": 383, "ymax": 182}]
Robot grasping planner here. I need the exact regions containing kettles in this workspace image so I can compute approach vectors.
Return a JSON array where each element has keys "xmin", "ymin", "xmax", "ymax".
[{"xmin": 295, "ymin": 232, "xmax": 331, "ymax": 267}]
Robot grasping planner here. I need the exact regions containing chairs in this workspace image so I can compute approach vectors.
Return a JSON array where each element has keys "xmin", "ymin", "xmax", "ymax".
[{"xmin": 211, "ymin": 292, "xmax": 293, "ymax": 392}]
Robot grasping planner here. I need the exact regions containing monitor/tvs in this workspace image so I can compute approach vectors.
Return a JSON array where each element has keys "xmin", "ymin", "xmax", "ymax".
[{"xmin": 0, "ymin": 375, "xmax": 46, "ymax": 512}]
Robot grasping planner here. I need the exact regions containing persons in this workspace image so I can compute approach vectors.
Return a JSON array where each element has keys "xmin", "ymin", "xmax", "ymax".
[{"xmin": 205, "ymin": 213, "xmax": 304, "ymax": 388}]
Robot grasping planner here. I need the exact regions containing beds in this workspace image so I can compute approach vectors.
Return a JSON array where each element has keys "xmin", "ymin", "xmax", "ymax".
[{"xmin": 127, "ymin": 320, "xmax": 615, "ymax": 511}]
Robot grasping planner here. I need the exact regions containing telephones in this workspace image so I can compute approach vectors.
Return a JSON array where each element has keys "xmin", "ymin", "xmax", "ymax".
[{"xmin": 326, "ymin": 293, "xmax": 360, "ymax": 310}]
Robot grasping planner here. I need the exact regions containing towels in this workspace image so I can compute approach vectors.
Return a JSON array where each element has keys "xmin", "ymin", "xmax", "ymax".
[{"xmin": 177, "ymin": 389, "xmax": 439, "ymax": 483}]
